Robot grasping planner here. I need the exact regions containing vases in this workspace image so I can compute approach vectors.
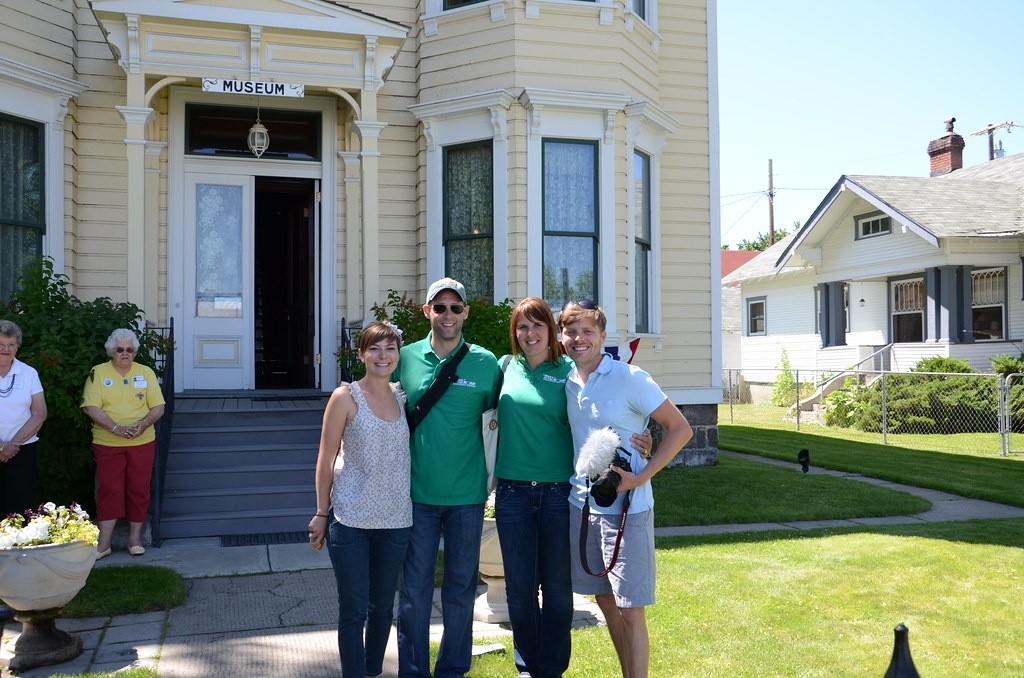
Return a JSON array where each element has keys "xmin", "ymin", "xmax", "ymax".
[{"xmin": 0, "ymin": 539, "xmax": 97, "ymax": 669}]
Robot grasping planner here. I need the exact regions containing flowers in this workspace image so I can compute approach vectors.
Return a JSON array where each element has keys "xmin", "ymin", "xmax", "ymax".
[{"xmin": 0, "ymin": 500, "xmax": 100, "ymax": 549}]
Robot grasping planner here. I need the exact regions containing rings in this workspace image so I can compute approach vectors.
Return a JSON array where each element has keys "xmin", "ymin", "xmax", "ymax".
[
  {"xmin": 644, "ymin": 449, "xmax": 647, "ymax": 453},
  {"xmin": 2, "ymin": 459, "xmax": 5, "ymax": 460},
  {"xmin": 124, "ymin": 433, "xmax": 126, "ymax": 434}
]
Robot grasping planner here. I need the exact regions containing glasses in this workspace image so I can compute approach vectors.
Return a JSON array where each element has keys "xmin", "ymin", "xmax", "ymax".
[
  {"xmin": 112, "ymin": 346, "xmax": 134, "ymax": 353},
  {"xmin": 431, "ymin": 303, "xmax": 464, "ymax": 315},
  {"xmin": 560, "ymin": 300, "xmax": 599, "ymax": 311},
  {"xmin": 0, "ymin": 341, "xmax": 16, "ymax": 349}
]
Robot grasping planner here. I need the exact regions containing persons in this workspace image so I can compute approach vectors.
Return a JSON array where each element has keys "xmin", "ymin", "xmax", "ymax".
[
  {"xmin": 552, "ymin": 300, "xmax": 693, "ymax": 678},
  {"xmin": 0, "ymin": 321, "xmax": 48, "ymax": 528},
  {"xmin": 79, "ymin": 328, "xmax": 166, "ymax": 563},
  {"xmin": 389, "ymin": 278, "xmax": 499, "ymax": 678},
  {"xmin": 496, "ymin": 298, "xmax": 654, "ymax": 678},
  {"xmin": 307, "ymin": 321, "xmax": 412, "ymax": 678}
]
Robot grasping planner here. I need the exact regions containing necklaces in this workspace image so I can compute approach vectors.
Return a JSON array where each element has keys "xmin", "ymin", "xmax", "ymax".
[{"xmin": 0, "ymin": 375, "xmax": 15, "ymax": 398}]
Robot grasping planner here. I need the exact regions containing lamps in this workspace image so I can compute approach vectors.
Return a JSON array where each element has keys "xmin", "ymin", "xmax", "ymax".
[
  {"xmin": 858, "ymin": 282, "xmax": 867, "ymax": 308},
  {"xmin": 247, "ymin": 95, "xmax": 270, "ymax": 159}
]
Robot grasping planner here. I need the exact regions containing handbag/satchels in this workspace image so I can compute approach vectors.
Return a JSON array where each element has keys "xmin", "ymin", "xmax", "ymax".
[{"xmin": 479, "ymin": 356, "xmax": 516, "ymax": 495}]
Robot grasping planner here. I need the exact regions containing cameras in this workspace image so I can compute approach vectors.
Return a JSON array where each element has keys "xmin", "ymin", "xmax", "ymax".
[{"xmin": 588, "ymin": 426, "xmax": 634, "ymax": 507}]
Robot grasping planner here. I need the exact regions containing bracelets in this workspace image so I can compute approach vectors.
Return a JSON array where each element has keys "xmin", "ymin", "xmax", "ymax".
[
  {"xmin": 314, "ymin": 515, "xmax": 329, "ymax": 518},
  {"xmin": 112, "ymin": 425, "xmax": 117, "ymax": 432},
  {"xmin": 144, "ymin": 419, "xmax": 149, "ymax": 427},
  {"xmin": 1, "ymin": 441, "xmax": 4, "ymax": 451}
]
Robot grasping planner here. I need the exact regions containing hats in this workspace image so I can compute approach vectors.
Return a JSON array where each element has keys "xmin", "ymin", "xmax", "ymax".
[{"xmin": 425, "ymin": 278, "xmax": 467, "ymax": 306}]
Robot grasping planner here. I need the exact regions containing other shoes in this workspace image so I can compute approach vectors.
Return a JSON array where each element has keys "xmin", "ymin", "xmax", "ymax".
[
  {"xmin": 95, "ymin": 548, "xmax": 111, "ymax": 561},
  {"xmin": 127, "ymin": 544, "xmax": 145, "ymax": 555}
]
